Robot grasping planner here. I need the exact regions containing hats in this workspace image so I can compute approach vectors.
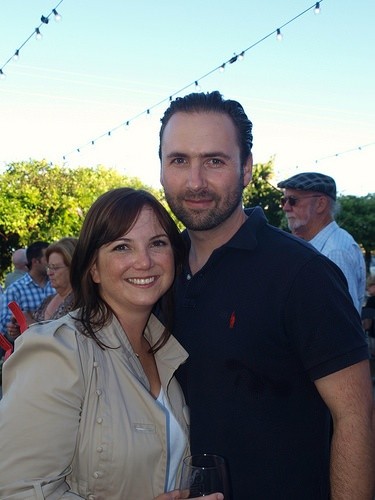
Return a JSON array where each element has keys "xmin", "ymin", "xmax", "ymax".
[{"xmin": 276, "ymin": 171, "xmax": 337, "ymax": 199}]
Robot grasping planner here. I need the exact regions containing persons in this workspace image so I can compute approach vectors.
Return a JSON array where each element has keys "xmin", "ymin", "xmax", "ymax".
[
  {"xmin": 277, "ymin": 173, "xmax": 375, "ymax": 361},
  {"xmin": 0, "ymin": 237, "xmax": 80, "ymax": 400},
  {"xmin": 0, "ymin": 187, "xmax": 224, "ymax": 500},
  {"xmin": 151, "ymin": 90, "xmax": 375, "ymax": 500}
]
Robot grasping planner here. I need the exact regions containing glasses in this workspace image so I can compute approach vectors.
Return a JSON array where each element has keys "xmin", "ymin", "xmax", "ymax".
[
  {"xmin": 44, "ymin": 264, "xmax": 67, "ymax": 272},
  {"xmin": 280, "ymin": 195, "xmax": 321, "ymax": 207}
]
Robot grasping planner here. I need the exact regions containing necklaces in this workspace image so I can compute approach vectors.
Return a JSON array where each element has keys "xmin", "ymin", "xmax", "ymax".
[{"xmin": 135, "ymin": 351, "xmax": 140, "ymax": 358}]
